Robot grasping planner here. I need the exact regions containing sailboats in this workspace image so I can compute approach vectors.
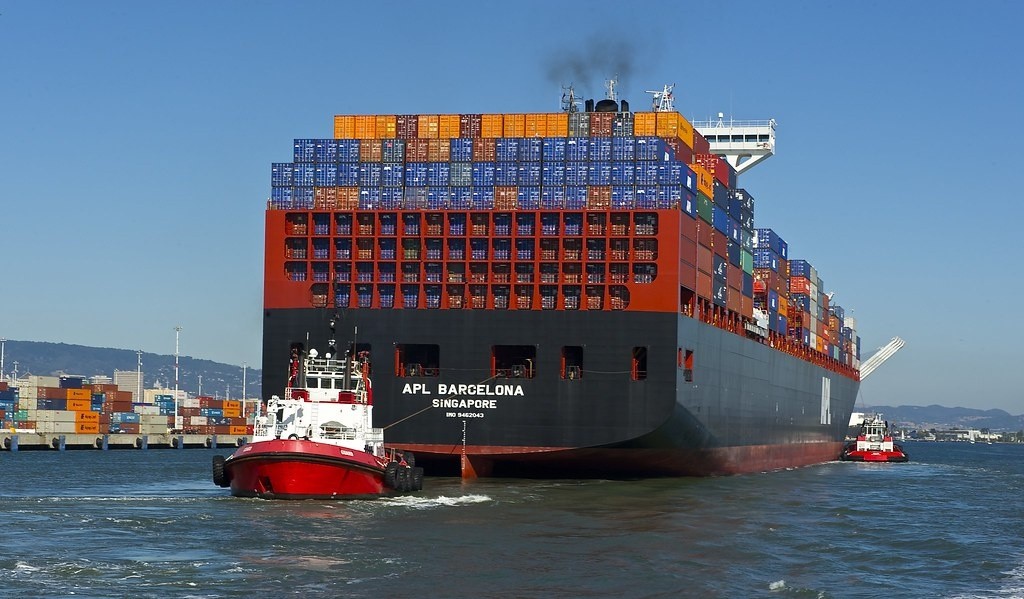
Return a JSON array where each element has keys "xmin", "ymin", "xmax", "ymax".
[
  {"xmin": 899, "ymin": 430, "xmax": 905, "ymax": 443},
  {"xmin": 969, "ymin": 429, "xmax": 975, "ymax": 444},
  {"xmin": 987, "ymin": 431, "xmax": 992, "ymax": 445}
]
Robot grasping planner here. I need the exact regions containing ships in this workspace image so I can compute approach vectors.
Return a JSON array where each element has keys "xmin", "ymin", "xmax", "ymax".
[{"xmin": 261, "ymin": 74, "xmax": 861, "ymax": 477}]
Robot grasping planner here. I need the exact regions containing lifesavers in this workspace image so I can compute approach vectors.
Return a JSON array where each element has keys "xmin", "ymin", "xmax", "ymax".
[
  {"xmin": 384, "ymin": 451, "xmax": 424, "ymax": 493},
  {"xmin": 212, "ymin": 454, "xmax": 230, "ymax": 488}
]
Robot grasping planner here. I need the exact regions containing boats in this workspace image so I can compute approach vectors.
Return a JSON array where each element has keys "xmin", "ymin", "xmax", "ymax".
[
  {"xmin": 212, "ymin": 313, "xmax": 424, "ymax": 501},
  {"xmin": 842, "ymin": 414, "xmax": 906, "ymax": 462}
]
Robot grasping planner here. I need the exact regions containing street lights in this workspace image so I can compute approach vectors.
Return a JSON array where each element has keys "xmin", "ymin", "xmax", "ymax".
[
  {"xmin": 215, "ymin": 391, "xmax": 219, "ymax": 400},
  {"xmin": 198, "ymin": 374, "xmax": 202, "ymax": 395},
  {"xmin": 0, "ymin": 338, "xmax": 7, "ymax": 381},
  {"xmin": 136, "ymin": 350, "xmax": 143, "ymax": 403},
  {"xmin": 240, "ymin": 361, "xmax": 248, "ymax": 418},
  {"xmin": 11, "ymin": 361, "xmax": 19, "ymax": 380},
  {"xmin": 174, "ymin": 326, "xmax": 184, "ymax": 430}
]
